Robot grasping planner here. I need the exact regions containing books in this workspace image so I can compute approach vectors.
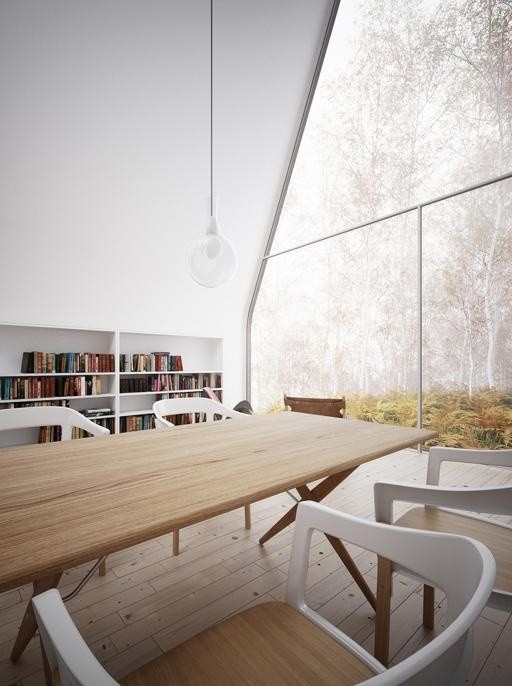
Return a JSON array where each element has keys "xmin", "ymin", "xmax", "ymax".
[{"xmin": 0, "ymin": 352, "xmax": 221, "ymax": 444}]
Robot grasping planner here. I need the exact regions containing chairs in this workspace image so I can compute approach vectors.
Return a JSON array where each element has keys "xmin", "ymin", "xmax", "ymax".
[
  {"xmin": 202, "ymin": 387, "xmax": 253, "ymax": 420},
  {"xmin": 153, "ymin": 397, "xmax": 254, "ymax": 555},
  {"xmin": 28, "ymin": 500, "xmax": 496, "ymax": 686},
  {"xmin": 282, "ymin": 392, "xmax": 349, "ymax": 421},
  {"xmin": 0, "ymin": 406, "xmax": 110, "ymax": 583},
  {"xmin": 374, "ymin": 445, "xmax": 512, "ymax": 667}
]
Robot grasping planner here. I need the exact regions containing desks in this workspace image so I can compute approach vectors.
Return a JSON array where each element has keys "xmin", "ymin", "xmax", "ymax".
[{"xmin": 0, "ymin": 411, "xmax": 439, "ymax": 686}]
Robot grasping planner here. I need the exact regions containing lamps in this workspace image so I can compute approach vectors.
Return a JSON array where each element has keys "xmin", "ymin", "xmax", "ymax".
[{"xmin": 187, "ymin": 0, "xmax": 237, "ymax": 289}]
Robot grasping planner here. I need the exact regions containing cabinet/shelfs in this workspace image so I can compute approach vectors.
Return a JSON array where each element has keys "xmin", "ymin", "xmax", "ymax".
[
  {"xmin": 117, "ymin": 330, "xmax": 224, "ymax": 432},
  {"xmin": 0, "ymin": 322, "xmax": 117, "ymax": 448}
]
